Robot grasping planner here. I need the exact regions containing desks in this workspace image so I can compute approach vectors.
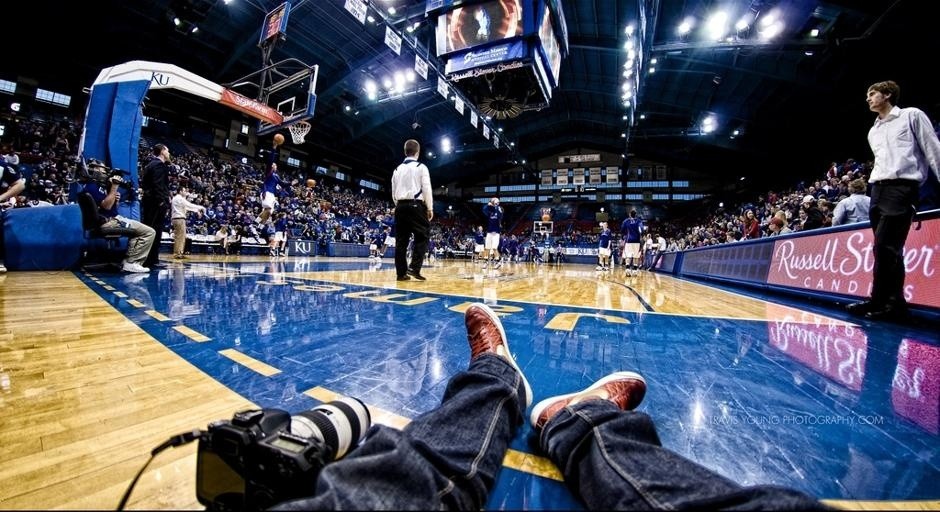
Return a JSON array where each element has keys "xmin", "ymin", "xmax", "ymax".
[
  {"xmin": 384, "ymin": 247, "xmax": 396, "ymax": 257},
  {"xmin": 288, "ymin": 240, "xmax": 319, "ymax": 256},
  {"xmin": 3, "ymin": 204, "xmax": 84, "ymax": 270},
  {"xmin": 326, "ymin": 243, "xmax": 370, "ymax": 257}
]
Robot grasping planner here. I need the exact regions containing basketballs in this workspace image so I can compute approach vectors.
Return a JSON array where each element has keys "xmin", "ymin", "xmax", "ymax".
[
  {"xmin": 273, "ymin": 133, "xmax": 284, "ymax": 145},
  {"xmin": 491, "ymin": 198, "xmax": 500, "ymax": 205},
  {"xmin": 543, "ymin": 214, "xmax": 550, "ymax": 221}
]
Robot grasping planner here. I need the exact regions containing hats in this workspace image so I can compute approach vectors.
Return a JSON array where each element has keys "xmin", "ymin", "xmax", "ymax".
[{"xmin": 799, "ymin": 194, "xmax": 815, "ymax": 206}]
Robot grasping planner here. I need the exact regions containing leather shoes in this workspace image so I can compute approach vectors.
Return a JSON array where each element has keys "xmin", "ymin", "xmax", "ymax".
[
  {"xmin": 148, "ymin": 263, "xmax": 168, "ymax": 268},
  {"xmin": 396, "ymin": 275, "xmax": 411, "ymax": 281},
  {"xmin": 864, "ymin": 305, "xmax": 911, "ymax": 321},
  {"xmin": 464, "ymin": 300, "xmax": 534, "ymax": 409},
  {"xmin": 845, "ymin": 299, "xmax": 876, "ymax": 316},
  {"xmin": 530, "ymin": 369, "xmax": 649, "ymax": 435},
  {"xmin": 406, "ymin": 266, "xmax": 426, "ymax": 281}
]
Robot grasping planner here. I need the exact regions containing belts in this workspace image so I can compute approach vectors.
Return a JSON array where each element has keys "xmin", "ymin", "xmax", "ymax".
[
  {"xmin": 396, "ymin": 203, "xmax": 420, "ymax": 208},
  {"xmin": 172, "ymin": 217, "xmax": 186, "ymax": 220},
  {"xmin": 875, "ymin": 179, "xmax": 920, "ymax": 187}
]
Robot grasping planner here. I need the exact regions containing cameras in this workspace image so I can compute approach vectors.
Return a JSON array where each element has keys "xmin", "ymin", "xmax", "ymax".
[{"xmin": 195, "ymin": 395, "xmax": 371, "ymax": 506}]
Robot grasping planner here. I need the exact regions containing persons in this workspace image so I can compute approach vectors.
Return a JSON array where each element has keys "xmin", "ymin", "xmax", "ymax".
[
  {"xmin": 649, "ymin": 233, "xmax": 668, "ymax": 273},
  {"xmin": 597, "ymin": 222, "xmax": 613, "ymax": 272},
  {"xmin": 266, "ymin": 303, "xmax": 849, "ymax": 511},
  {"xmin": 482, "ymin": 198, "xmax": 505, "ymax": 271},
  {"xmin": 83, "ymin": 160, "xmax": 157, "ymax": 274},
  {"xmin": 391, "ymin": 139, "xmax": 434, "ymax": 283},
  {"xmin": 621, "ymin": 210, "xmax": 645, "ymax": 278},
  {"xmin": 2, "ymin": 107, "xmax": 486, "ymax": 266},
  {"xmin": 847, "ymin": 80, "xmax": 940, "ymax": 322},
  {"xmin": 498, "ymin": 120, "xmax": 873, "ymax": 265}
]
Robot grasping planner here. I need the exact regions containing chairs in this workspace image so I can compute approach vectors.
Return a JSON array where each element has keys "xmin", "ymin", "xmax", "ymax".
[{"xmin": 77, "ymin": 192, "xmax": 136, "ymax": 274}]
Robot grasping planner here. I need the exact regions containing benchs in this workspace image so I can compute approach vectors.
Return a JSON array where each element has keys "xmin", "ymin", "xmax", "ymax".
[{"xmin": 162, "ymin": 231, "xmax": 270, "ymax": 254}]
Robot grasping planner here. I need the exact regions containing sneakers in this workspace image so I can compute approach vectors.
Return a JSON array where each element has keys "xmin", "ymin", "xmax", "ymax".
[
  {"xmin": 492, "ymin": 256, "xmax": 503, "ymax": 270},
  {"xmin": 247, "ymin": 223, "xmax": 259, "ymax": 237},
  {"xmin": 631, "ymin": 270, "xmax": 637, "ymax": 277},
  {"xmin": 625, "ymin": 268, "xmax": 631, "ymax": 277},
  {"xmin": 121, "ymin": 259, "xmax": 151, "ymax": 273},
  {"xmin": 266, "ymin": 250, "xmax": 286, "ymax": 257},
  {"xmin": 481, "ymin": 259, "xmax": 490, "ymax": 269},
  {"xmin": 253, "ymin": 231, "xmax": 261, "ymax": 244},
  {"xmin": 0, "ymin": 263, "xmax": 7, "ymax": 273},
  {"xmin": 596, "ymin": 265, "xmax": 609, "ymax": 271}
]
